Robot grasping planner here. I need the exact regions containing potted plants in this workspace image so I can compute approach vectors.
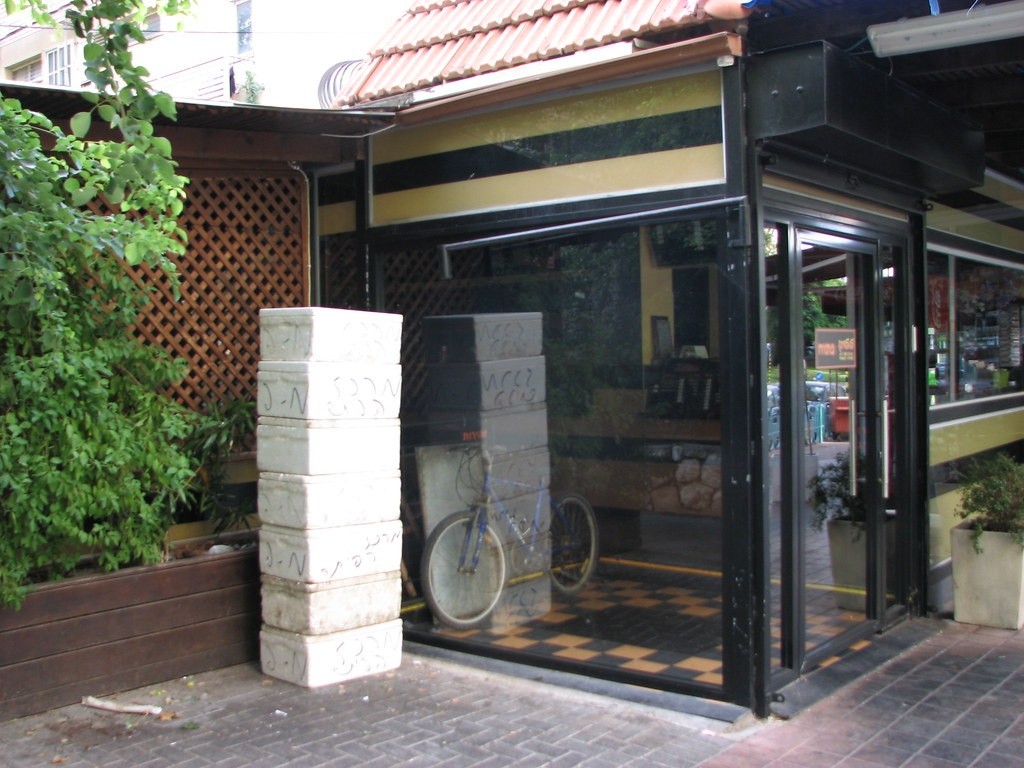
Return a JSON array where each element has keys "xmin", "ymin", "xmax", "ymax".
[
  {"xmin": 804, "ymin": 448, "xmax": 897, "ymax": 614},
  {"xmin": 950, "ymin": 450, "xmax": 1024, "ymax": 633}
]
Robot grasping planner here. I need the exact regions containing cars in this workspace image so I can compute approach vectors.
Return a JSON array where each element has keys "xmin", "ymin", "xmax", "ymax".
[{"xmin": 761, "ymin": 336, "xmax": 996, "ymax": 446}]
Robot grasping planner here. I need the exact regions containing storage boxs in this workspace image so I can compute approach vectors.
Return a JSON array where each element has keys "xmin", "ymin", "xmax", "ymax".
[
  {"xmin": 422, "ymin": 310, "xmax": 550, "ymax": 625},
  {"xmin": 255, "ymin": 307, "xmax": 403, "ymax": 689}
]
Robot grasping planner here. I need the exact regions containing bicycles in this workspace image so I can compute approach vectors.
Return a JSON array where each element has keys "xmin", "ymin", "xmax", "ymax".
[{"xmin": 420, "ymin": 439, "xmax": 602, "ymax": 631}]
